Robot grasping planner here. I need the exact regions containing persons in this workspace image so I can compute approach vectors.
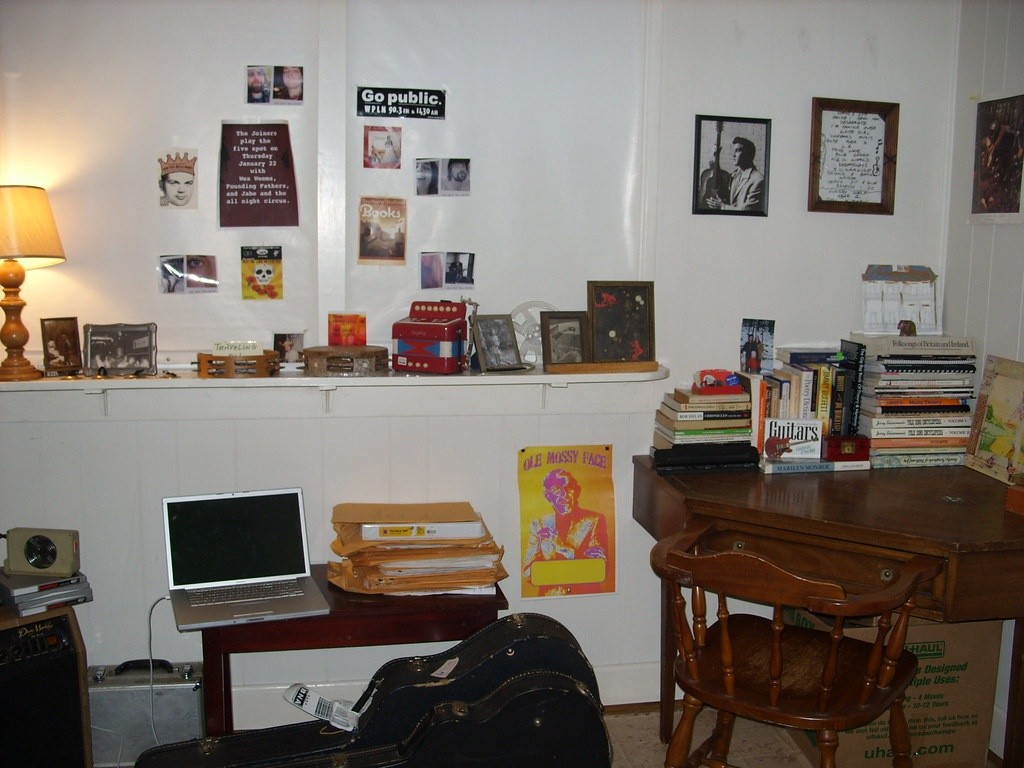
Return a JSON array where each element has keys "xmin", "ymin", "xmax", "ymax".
[
  {"xmin": 740, "ymin": 334, "xmax": 765, "ymax": 374},
  {"xmin": 370, "ymin": 136, "xmax": 398, "ymax": 165},
  {"xmin": 187, "ymin": 255, "xmax": 216, "ymax": 288},
  {"xmin": 60, "ymin": 334, "xmax": 76, "ymax": 368},
  {"xmin": 248, "ymin": 66, "xmax": 302, "ymax": 104},
  {"xmin": 422, "ymin": 252, "xmax": 441, "ymax": 288},
  {"xmin": 707, "ymin": 137, "xmax": 766, "ymax": 212},
  {"xmin": 416, "ymin": 158, "xmax": 470, "ymax": 195},
  {"xmin": 93, "ymin": 353, "xmax": 149, "ymax": 369},
  {"xmin": 488, "ymin": 329, "xmax": 504, "ymax": 367},
  {"xmin": 449, "ymin": 255, "xmax": 465, "ymax": 285}
]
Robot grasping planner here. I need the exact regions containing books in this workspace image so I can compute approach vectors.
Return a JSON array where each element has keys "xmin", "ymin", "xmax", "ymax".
[{"xmin": 650, "ymin": 332, "xmax": 980, "ymax": 473}]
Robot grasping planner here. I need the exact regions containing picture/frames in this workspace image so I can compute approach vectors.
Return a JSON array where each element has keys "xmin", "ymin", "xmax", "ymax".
[
  {"xmin": 540, "ymin": 311, "xmax": 589, "ymax": 372},
  {"xmin": 807, "ymin": 97, "xmax": 901, "ymax": 215},
  {"xmin": 40, "ymin": 317, "xmax": 83, "ymax": 372},
  {"xmin": 468, "ymin": 314, "xmax": 522, "ymax": 374},
  {"xmin": 83, "ymin": 322, "xmax": 158, "ymax": 376},
  {"xmin": 963, "ymin": 354, "xmax": 1024, "ymax": 485},
  {"xmin": 586, "ymin": 280, "xmax": 656, "ymax": 363},
  {"xmin": 691, "ymin": 114, "xmax": 772, "ymax": 217}
]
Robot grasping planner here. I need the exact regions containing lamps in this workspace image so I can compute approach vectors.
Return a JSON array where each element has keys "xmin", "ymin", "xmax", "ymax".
[{"xmin": 0, "ymin": 184, "xmax": 66, "ymax": 381}]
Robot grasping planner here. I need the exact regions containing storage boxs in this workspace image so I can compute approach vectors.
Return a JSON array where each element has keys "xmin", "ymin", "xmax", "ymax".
[{"xmin": 773, "ymin": 607, "xmax": 1003, "ymax": 768}]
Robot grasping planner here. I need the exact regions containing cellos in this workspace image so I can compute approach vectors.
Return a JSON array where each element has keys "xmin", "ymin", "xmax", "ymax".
[{"xmin": 697, "ymin": 121, "xmax": 730, "ymax": 209}]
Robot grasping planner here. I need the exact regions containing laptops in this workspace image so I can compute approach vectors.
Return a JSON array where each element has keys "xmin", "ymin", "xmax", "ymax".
[{"xmin": 162, "ymin": 486, "xmax": 330, "ymax": 631}]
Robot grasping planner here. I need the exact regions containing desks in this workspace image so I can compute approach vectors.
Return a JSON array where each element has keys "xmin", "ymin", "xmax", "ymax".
[
  {"xmin": 201, "ymin": 564, "xmax": 509, "ymax": 738},
  {"xmin": 631, "ymin": 455, "xmax": 1024, "ymax": 768}
]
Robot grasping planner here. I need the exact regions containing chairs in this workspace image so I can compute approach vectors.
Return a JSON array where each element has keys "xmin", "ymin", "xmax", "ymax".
[{"xmin": 649, "ymin": 520, "xmax": 943, "ymax": 768}]
